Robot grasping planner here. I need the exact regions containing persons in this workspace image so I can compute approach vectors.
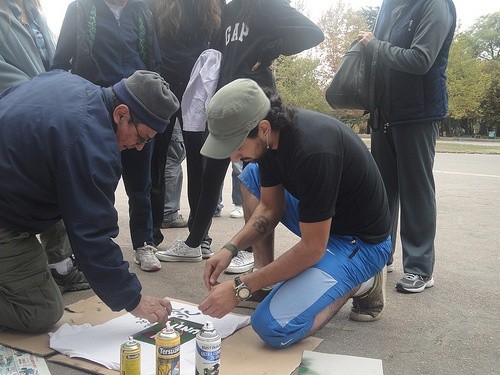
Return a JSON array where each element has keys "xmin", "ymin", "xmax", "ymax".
[
  {"xmin": 51, "ymin": 0, "xmax": 166, "ymax": 271},
  {"xmin": 197, "ymin": 77, "xmax": 393, "ymax": 348},
  {"xmin": 151, "ymin": 0, "xmax": 325, "ymax": 274},
  {"xmin": 0, "ymin": 68, "xmax": 172, "ymax": 335},
  {"xmin": 0, "ymin": 0, "xmax": 57, "ymax": 93},
  {"xmin": 357, "ymin": 0, "xmax": 456, "ymax": 293}
]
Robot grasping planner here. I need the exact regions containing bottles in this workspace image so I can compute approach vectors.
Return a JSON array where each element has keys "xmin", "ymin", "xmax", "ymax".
[
  {"xmin": 196, "ymin": 320, "xmax": 222, "ymax": 375},
  {"xmin": 120, "ymin": 336, "xmax": 141, "ymax": 375},
  {"xmin": 155, "ymin": 320, "xmax": 180, "ymax": 375}
]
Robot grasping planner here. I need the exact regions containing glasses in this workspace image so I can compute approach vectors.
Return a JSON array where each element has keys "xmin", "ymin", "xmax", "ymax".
[{"xmin": 131, "ymin": 113, "xmax": 152, "ymax": 145}]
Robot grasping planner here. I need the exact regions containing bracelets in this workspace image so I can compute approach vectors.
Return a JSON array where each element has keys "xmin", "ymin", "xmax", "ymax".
[{"xmin": 222, "ymin": 243, "xmax": 238, "ymax": 258}]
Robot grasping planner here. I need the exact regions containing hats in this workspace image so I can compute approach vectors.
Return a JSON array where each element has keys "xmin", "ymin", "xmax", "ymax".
[
  {"xmin": 200, "ymin": 77, "xmax": 272, "ymax": 159},
  {"xmin": 112, "ymin": 69, "xmax": 180, "ymax": 135}
]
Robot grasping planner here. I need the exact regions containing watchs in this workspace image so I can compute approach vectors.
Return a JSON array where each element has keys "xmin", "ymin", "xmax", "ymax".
[{"xmin": 234, "ymin": 276, "xmax": 252, "ymax": 301}]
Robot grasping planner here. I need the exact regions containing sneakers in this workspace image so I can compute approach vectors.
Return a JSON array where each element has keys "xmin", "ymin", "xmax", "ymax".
[
  {"xmin": 229, "ymin": 205, "xmax": 244, "ymax": 218},
  {"xmin": 222, "ymin": 251, "xmax": 255, "ymax": 274},
  {"xmin": 394, "ymin": 271, "xmax": 435, "ymax": 292},
  {"xmin": 134, "ymin": 242, "xmax": 162, "ymax": 272},
  {"xmin": 386, "ymin": 262, "xmax": 395, "ymax": 274},
  {"xmin": 154, "ymin": 239, "xmax": 204, "ymax": 263},
  {"xmin": 49, "ymin": 254, "xmax": 92, "ymax": 294}
]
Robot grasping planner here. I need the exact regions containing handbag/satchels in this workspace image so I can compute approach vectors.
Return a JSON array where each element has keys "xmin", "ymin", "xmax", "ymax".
[{"xmin": 323, "ymin": 38, "xmax": 388, "ymax": 112}]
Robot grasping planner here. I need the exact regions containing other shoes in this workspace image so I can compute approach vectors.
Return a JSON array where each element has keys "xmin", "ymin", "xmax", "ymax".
[
  {"xmin": 234, "ymin": 267, "xmax": 278, "ymax": 310},
  {"xmin": 200, "ymin": 240, "xmax": 214, "ymax": 260},
  {"xmin": 349, "ymin": 264, "xmax": 388, "ymax": 322},
  {"xmin": 213, "ymin": 210, "xmax": 221, "ymax": 217},
  {"xmin": 160, "ymin": 210, "xmax": 188, "ymax": 228}
]
